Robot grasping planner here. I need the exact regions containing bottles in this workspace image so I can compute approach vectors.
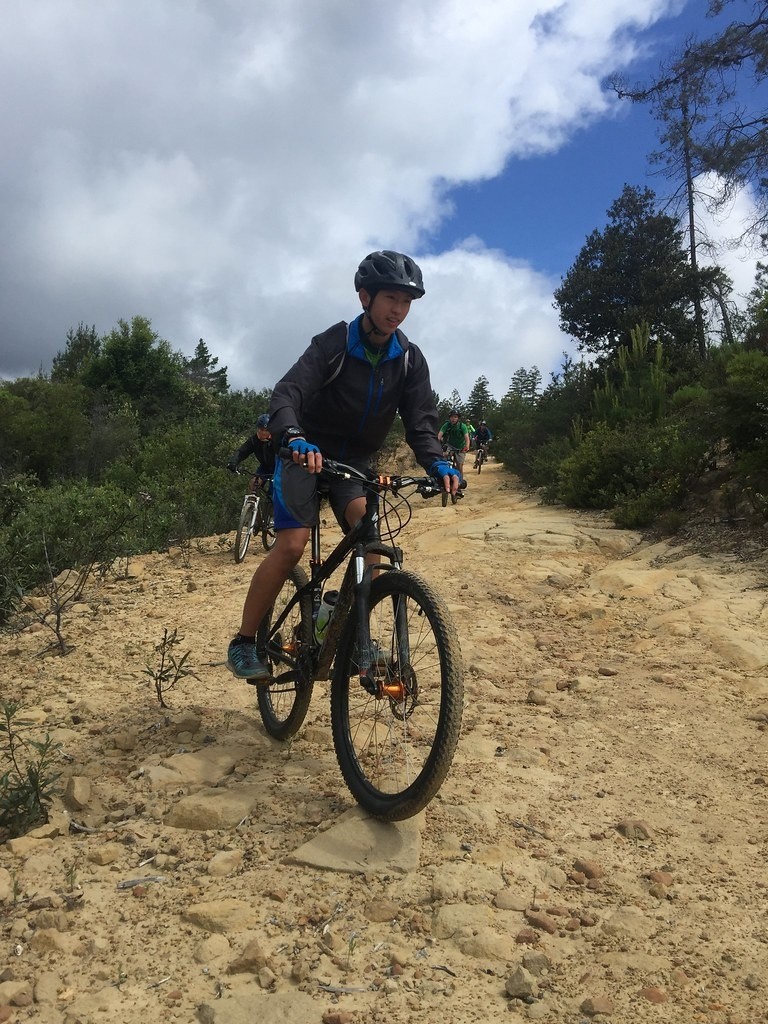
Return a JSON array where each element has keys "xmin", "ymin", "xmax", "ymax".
[{"xmin": 314, "ymin": 590, "xmax": 339, "ymax": 645}]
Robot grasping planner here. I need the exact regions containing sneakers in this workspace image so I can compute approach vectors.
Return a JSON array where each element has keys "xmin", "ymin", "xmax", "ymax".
[
  {"xmin": 225, "ymin": 639, "xmax": 265, "ymax": 680},
  {"xmin": 350, "ymin": 639, "xmax": 395, "ymax": 676}
]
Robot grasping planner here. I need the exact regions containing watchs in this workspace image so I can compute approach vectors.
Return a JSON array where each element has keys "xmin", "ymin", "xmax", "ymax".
[{"xmin": 283, "ymin": 427, "xmax": 306, "ymax": 448}]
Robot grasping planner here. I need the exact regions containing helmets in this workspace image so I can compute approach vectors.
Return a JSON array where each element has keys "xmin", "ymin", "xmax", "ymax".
[
  {"xmin": 354, "ymin": 250, "xmax": 425, "ymax": 299},
  {"xmin": 256, "ymin": 414, "xmax": 270, "ymax": 428},
  {"xmin": 448, "ymin": 409, "xmax": 461, "ymax": 417}
]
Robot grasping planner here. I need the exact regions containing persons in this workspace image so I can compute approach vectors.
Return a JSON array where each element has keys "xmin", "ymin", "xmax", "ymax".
[
  {"xmin": 224, "ymin": 250, "xmax": 462, "ymax": 678},
  {"xmin": 438, "ymin": 410, "xmax": 493, "ymax": 498},
  {"xmin": 226, "ymin": 414, "xmax": 275, "ymax": 529}
]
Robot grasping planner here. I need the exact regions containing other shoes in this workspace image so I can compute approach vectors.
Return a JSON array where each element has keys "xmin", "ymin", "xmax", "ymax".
[
  {"xmin": 473, "ymin": 465, "xmax": 477, "ymax": 469},
  {"xmin": 254, "ymin": 520, "xmax": 262, "ymax": 537},
  {"xmin": 484, "ymin": 457, "xmax": 488, "ymax": 461}
]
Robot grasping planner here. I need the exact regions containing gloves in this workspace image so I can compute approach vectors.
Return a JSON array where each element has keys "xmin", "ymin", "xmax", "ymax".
[{"xmin": 227, "ymin": 465, "xmax": 240, "ymax": 473}]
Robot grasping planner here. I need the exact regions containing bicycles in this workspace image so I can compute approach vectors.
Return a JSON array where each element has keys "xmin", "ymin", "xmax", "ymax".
[
  {"xmin": 234, "ymin": 467, "xmax": 278, "ymax": 563},
  {"xmin": 474, "ymin": 438, "xmax": 490, "ymax": 475},
  {"xmin": 442, "ymin": 445, "xmax": 466, "ymax": 507},
  {"xmin": 245, "ymin": 446, "xmax": 468, "ymax": 822}
]
